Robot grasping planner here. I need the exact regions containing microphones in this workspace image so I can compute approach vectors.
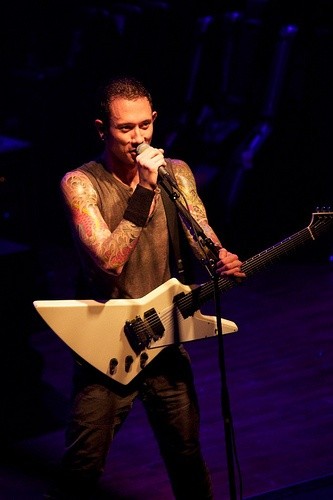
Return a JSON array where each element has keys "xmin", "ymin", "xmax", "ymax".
[{"xmin": 136, "ymin": 143, "xmax": 170, "ymax": 178}]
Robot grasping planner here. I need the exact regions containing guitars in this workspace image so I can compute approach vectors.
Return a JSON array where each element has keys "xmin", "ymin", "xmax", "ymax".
[{"xmin": 34, "ymin": 207, "xmax": 332, "ymax": 385}]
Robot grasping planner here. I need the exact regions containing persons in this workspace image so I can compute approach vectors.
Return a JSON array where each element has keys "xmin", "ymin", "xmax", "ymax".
[{"xmin": 60, "ymin": 75, "xmax": 247, "ymax": 500}]
